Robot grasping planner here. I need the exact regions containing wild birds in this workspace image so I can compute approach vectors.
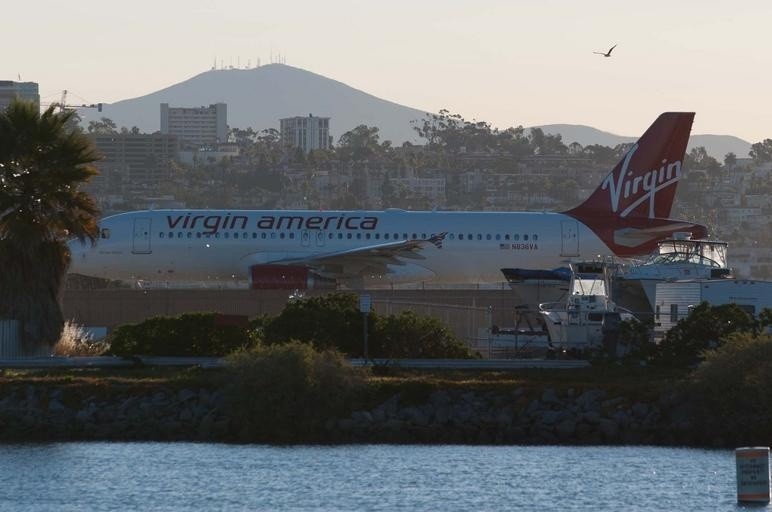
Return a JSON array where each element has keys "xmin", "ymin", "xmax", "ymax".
[{"xmin": 603, "ymin": 46, "xmax": 616, "ymax": 57}]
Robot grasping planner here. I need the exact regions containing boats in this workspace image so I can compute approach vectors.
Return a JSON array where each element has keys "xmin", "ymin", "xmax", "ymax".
[
  {"xmin": 536, "ymin": 293, "xmax": 652, "ymax": 356},
  {"xmin": 498, "ymin": 230, "xmax": 733, "ymax": 324},
  {"xmin": 485, "ymin": 323, "xmax": 550, "ymax": 359}
]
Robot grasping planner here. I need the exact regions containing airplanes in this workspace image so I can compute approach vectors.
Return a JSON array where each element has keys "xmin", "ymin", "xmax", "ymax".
[{"xmin": 63, "ymin": 111, "xmax": 708, "ymax": 290}]
